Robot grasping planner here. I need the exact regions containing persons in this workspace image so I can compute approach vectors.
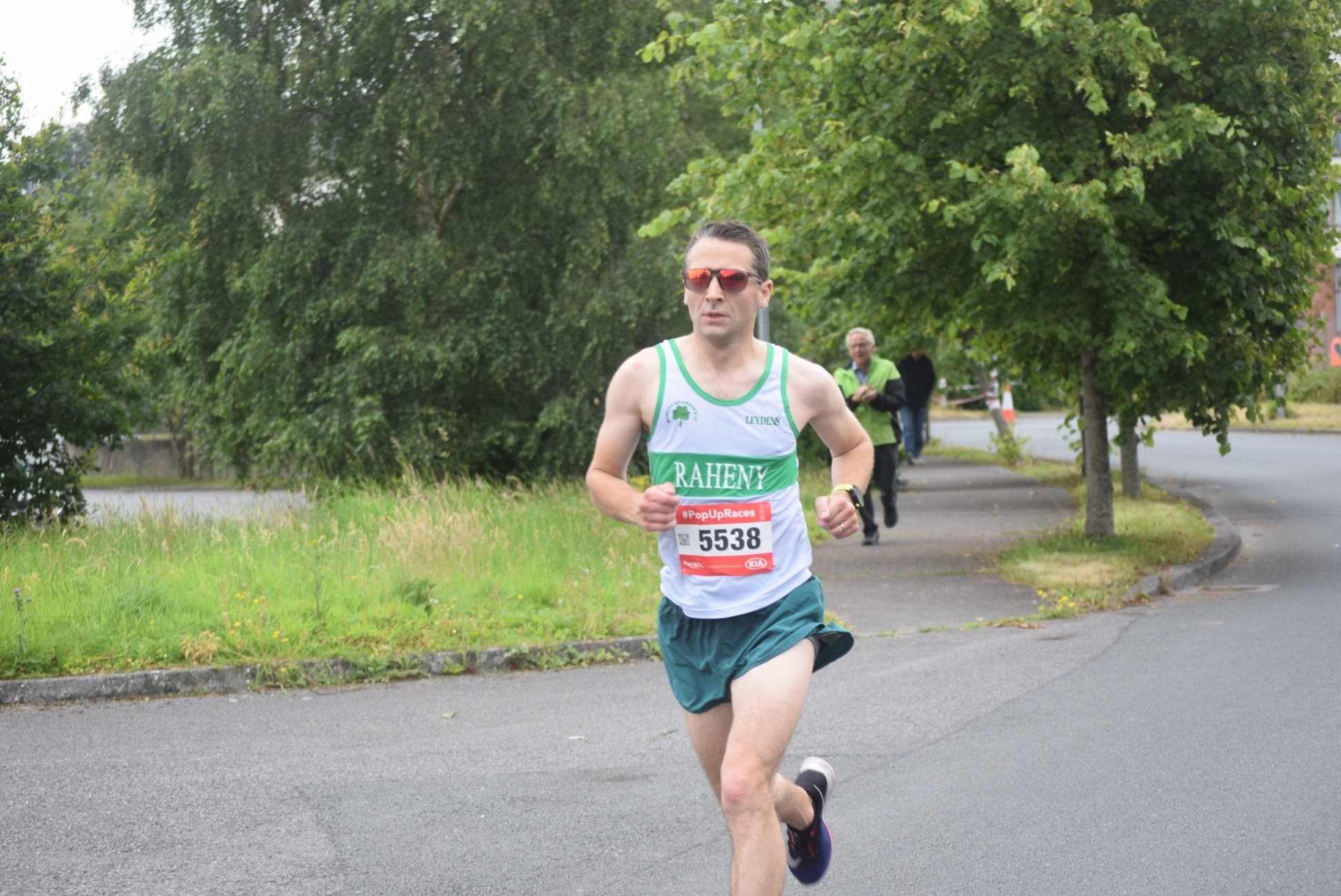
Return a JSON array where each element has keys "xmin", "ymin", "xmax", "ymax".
[
  {"xmin": 895, "ymin": 341, "xmax": 937, "ymax": 466},
  {"xmin": 585, "ymin": 223, "xmax": 875, "ymax": 896},
  {"xmin": 832, "ymin": 327, "xmax": 907, "ymax": 548}
]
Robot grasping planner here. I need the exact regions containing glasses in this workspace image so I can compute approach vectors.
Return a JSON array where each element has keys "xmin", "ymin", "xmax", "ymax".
[{"xmin": 681, "ymin": 268, "xmax": 764, "ymax": 294}]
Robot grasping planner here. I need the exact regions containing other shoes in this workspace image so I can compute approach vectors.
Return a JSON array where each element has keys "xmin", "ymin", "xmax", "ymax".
[{"xmin": 908, "ymin": 453, "xmax": 924, "ymax": 465}]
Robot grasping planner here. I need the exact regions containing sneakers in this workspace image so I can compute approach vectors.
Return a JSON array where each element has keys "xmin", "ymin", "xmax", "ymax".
[
  {"xmin": 861, "ymin": 532, "xmax": 880, "ymax": 546},
  {"xmin": 781, "ymin": 755, "xmax": 835, "ymax": 886},
  {"xmin": 884, "ymin": 509, "xmax": 898, "ymax": 528}
]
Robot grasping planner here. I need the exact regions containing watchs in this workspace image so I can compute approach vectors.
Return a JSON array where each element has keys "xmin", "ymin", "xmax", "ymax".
[{"xmin": 830, "ymin": 484, "xmax": 864, "ymax": 510}]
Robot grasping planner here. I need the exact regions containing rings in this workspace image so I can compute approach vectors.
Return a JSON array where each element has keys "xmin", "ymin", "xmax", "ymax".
[{"xmin": 839, "ymin": 523, "xmax": 845, "ymax": 532}]
